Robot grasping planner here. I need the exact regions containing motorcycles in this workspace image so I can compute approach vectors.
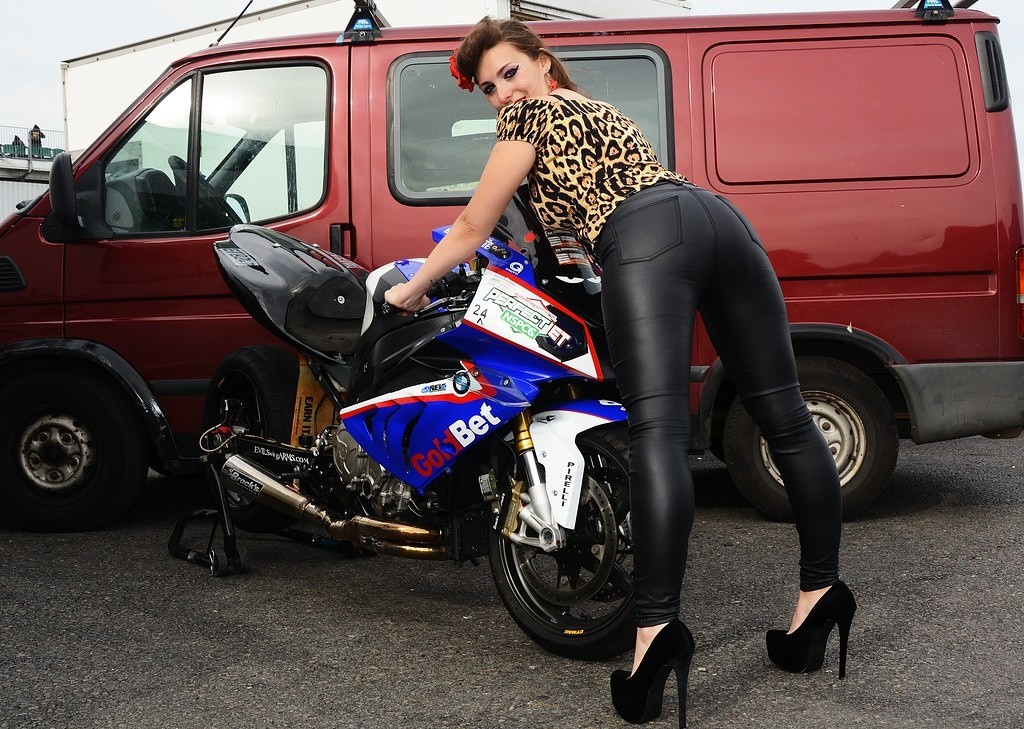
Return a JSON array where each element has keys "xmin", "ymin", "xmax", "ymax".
[{"xmin": 165, "ymin": 220, "xmax": 642, "ymax": 662}]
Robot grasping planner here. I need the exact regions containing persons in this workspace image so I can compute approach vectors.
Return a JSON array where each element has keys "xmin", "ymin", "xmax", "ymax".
[{"xmin": 383, "ymin": 14, "xmax": 857, "ymax": 729}]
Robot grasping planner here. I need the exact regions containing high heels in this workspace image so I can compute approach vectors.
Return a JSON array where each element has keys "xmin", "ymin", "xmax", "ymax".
[
  {"xmin": 609, "ymin": 618, "xmax": 696, "ymax": 729},
  {"xmin": 766, "ymin": 580, "xmax": 856, "ymax": 680}
]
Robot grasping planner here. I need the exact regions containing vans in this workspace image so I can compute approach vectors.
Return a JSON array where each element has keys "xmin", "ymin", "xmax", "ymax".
[{"xmin": 0, "ymin": 0, "xmax": 1024, "ymax": 526}]
